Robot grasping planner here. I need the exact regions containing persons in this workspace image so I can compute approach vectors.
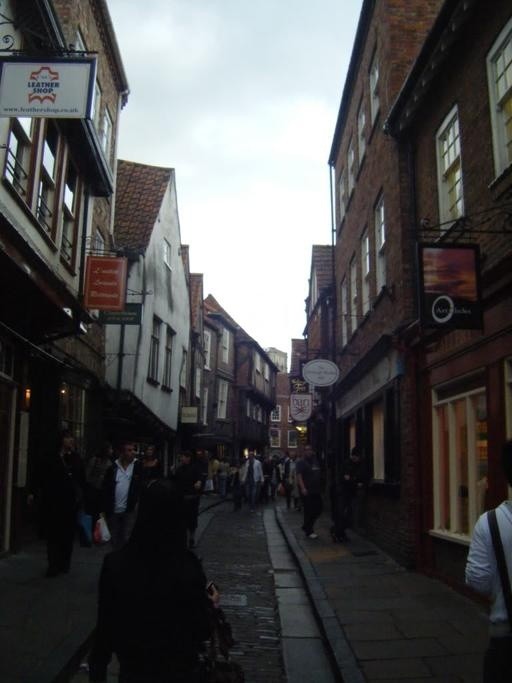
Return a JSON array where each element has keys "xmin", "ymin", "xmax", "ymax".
[
  {"xmin": 331, "ymin": 447, "xmax": 366, "ymax": 544},
  {"xmin": 81, "ymin": 442, "xmax": 113, "ymax": 548},
  {"xmin": 177, "ymin": 451, "xmax": 204, "ymax": 544},
  {"xmin": 196, "ymin": 451, "xmax": 303, "ymax": 513},
  {"xmin": 100, "ymin": 441, "xmax": 142, "ymax": 553},
  {"xmin": 40, "ymin": 431, "xmax": 85, "ymax": 577},
  {"xmin": 87, "ymin": 476, "xmax": 222, "ymax": 681},
  {"xmin": 132, "ymin": 444, "xmax": 164, "ymax": 517},
  {"xmin": 296, "ymin": 445, "xmax": 323, "ymax": 539},
  {"xmin": 462, "ymin": 437, "xmax": 512, "ymax": 682}
]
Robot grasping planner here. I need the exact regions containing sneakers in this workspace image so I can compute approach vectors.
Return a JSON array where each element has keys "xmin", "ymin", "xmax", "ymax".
[{"xmin": 309, "ymin": 532, "xmax": 318, "ymax": 538}]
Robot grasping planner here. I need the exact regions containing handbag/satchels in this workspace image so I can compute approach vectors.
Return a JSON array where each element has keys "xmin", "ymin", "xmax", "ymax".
[
  {"xmin": 200, "ymin": 607, "xmax": 246, "ymax": 682},
  {"xmin": 94, "ymin": 516, "xmax": 111, "ymax": 542}
]
